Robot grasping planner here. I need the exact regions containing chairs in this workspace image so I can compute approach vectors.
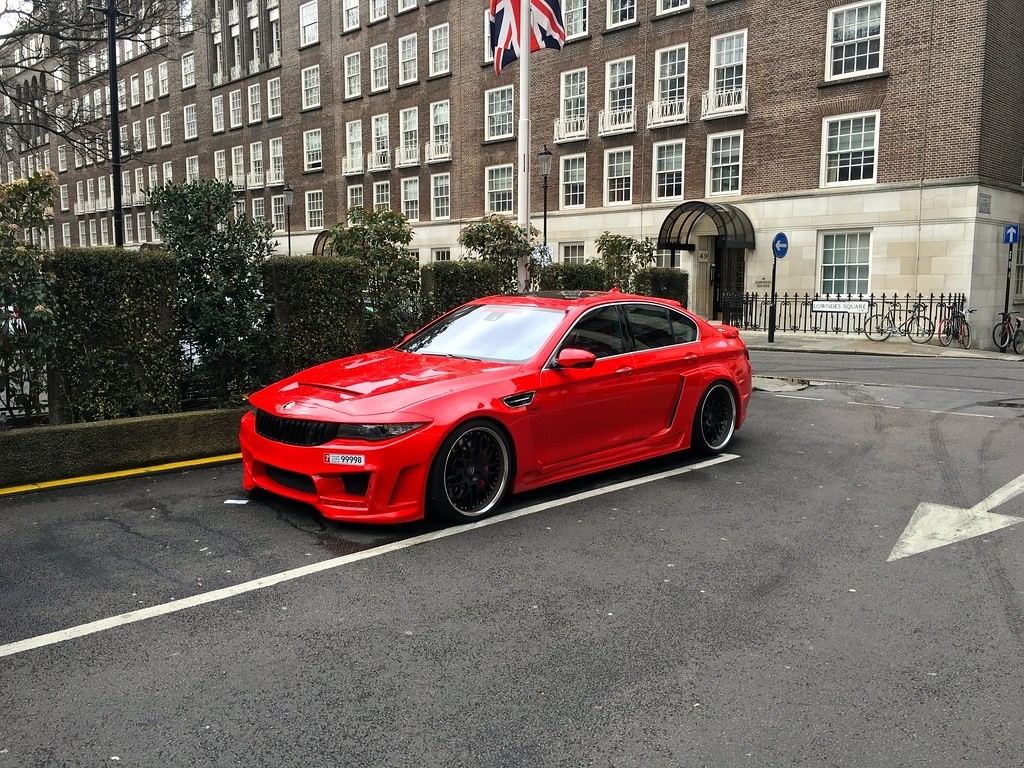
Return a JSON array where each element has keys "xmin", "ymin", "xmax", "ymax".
[{"xmin": 574, "ymin": 311, "xmax": 615, "ymax": 358}]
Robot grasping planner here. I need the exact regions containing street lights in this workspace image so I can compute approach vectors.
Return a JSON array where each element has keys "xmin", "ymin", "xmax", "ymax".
[
  {"xmin": 538, "ymin": 142, "xmax": 553, "ymax": 257},
  {"xmin": 283, "ymin": 182, "xmax": 295, "ymax": 259}
]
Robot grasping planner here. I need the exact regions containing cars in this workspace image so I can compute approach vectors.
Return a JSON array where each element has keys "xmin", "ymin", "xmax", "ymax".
[{"xmin": 239, "ymin": 290, "xmax": 754, "ymax": 527}]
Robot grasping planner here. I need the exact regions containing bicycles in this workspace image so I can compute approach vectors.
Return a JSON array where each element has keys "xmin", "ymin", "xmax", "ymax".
[
  {"xmin": 940, "ymin": 306, "xmax": 978, "ymax": 350},
  {"xmin": 862, "ymin": 301, "xmax": 937, "ymax": 344},
  {"xmin": 993, "ymin": 310, "xmax": 1024, "ymax": 354}
]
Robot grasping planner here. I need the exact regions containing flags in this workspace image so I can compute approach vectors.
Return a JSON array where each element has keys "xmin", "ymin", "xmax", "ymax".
[{"xmin": 490, "ymin": 0, "xmax": 565, "ymax": 76}]
